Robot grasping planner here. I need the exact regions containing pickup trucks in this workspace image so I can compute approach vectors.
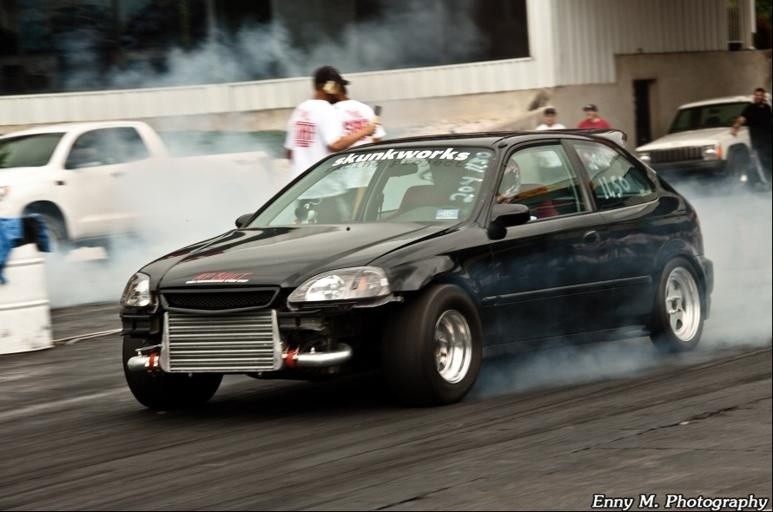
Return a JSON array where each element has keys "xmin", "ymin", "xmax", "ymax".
[{"xmin": 0, "ymin": 116, "xmax": 276, "ymax": 263}]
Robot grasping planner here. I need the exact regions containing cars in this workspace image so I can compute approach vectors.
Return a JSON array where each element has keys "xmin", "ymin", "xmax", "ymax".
[{"xmin": 115, "ymin": 128, "xmax": 716, "ymax": 412}]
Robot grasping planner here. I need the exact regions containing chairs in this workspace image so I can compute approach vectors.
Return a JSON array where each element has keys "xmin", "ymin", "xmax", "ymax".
[{"xmin": 516, "ymin": 184, "xmax": 561, "ymax": 218}]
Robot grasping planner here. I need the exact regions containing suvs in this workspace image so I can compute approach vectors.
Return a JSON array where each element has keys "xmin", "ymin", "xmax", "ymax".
[{"xmin": 634, "ymin": 92, "xmax": 773, "ymax": 192}]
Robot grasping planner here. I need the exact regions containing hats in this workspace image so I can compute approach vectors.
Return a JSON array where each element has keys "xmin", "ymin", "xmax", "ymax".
[
  {"xmin": 316, "ymin": 66, "xmax": 347, "ymax": 86},
  {"xmin": 583, "ymin": 104, "xmax": 597, "ymax": 111}
]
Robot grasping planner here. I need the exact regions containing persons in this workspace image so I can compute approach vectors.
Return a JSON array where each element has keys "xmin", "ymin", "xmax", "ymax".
[
  {"xmin": 281, "ymin": 66, "xmax": 377, "ymax": 172},
  {"xmin": 729, "ymin": 89, "xmax": 772, "ymax": 195},
  {"xmin": 529, "ymin": 104, "xmax": 568, "ymax": 183},
  {"xmin": 330, "ymin": 78, "xmax": 385, "ymax": 155},
  {"xmin": 575, "ymin": 102, "xmax": 614, "ymax": 131}
]
